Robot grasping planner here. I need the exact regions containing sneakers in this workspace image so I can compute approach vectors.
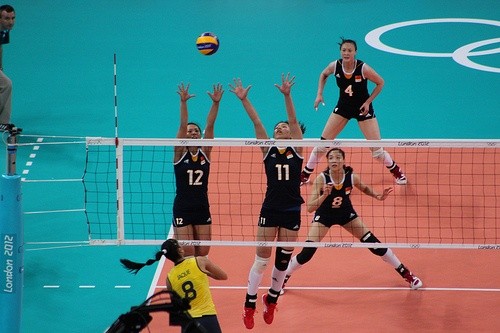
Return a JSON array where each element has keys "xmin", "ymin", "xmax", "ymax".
[
  {"xmin": 242, "ymin": 301, "xmax": 255, "ymax": 330},
  {"xmin": 402, "ymin": 269, "xmax": 423, "ymax": 289},
  {"xmin": 299, "ymin": 172, "xmax": 310, "ymax": 186},
  {"xmin": 0, "ymin": 124, "xmax": 23, "ymax": 134},
  {"xmin": 280, "ymin": 274, "xmax": 292, "ymax": 295},
  {"xmin": 262, "ymin": 294, "xmax": 279, "ymax": 324},
  {"xmin": 391, "ymin": 166, "xmax": 407, "ymax": 184}
]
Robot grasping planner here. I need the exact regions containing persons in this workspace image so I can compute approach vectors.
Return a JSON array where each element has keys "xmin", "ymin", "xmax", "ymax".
[
  {"xmin": 121, "ymin": 239, "xmax": 228, "ymax": 333},
  {"xmin": 227, "ymin": 73, "xmax": 306, "ymax": 329},
  {"xmin": 172, "ymin": 82, "xmax": 225, "ymax": 256},
  {"xmin": 279, "ymin": 148, "xmax": 424, "ymax": 295},
  {"xmin": 300, "ymin": 36, "xmax": 407, "ymax": 186},
  {"xmin": 0, "ymin": 5, "xmax": 22, "ymax": 134}
]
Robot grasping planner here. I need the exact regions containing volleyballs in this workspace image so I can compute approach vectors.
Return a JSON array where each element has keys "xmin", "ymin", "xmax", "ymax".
[{"xmin": 196, "ymin": 31, "xmax": 220, "ymax": 56}]
